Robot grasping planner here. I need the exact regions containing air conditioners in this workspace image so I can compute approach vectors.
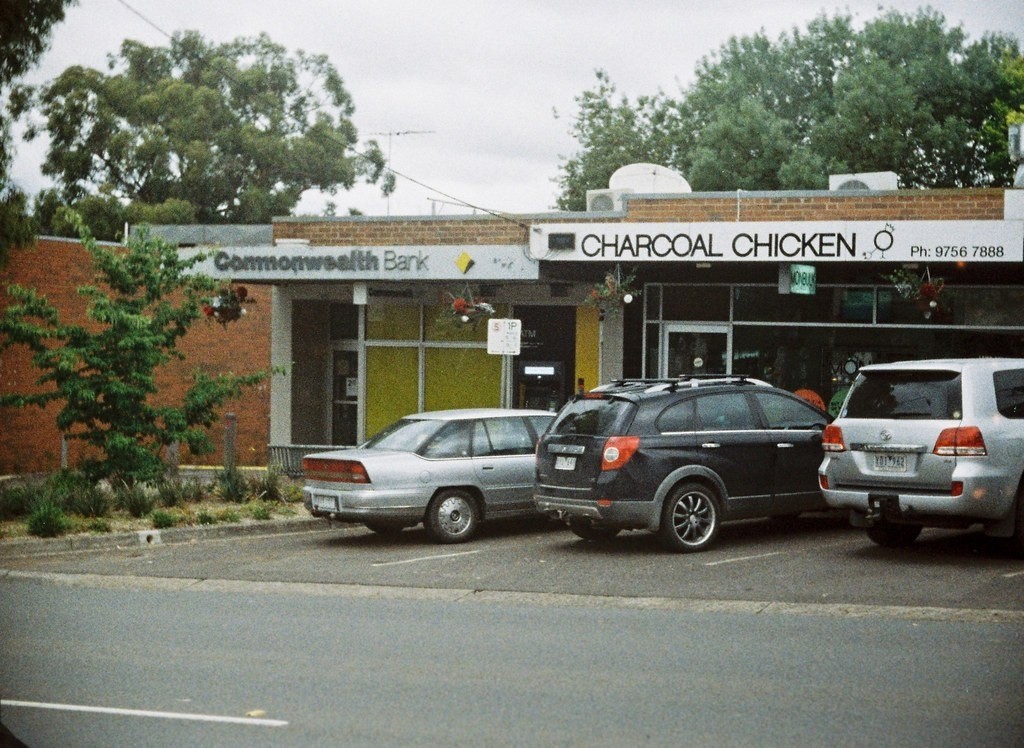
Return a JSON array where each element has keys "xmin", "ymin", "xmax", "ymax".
[
  {"xmin": 586, "ymin": 189, "xmax": 633, "ymax": 212},
  {"xmin": 829, "ymin": 170, "xmax": 898, "ymax": 190}
]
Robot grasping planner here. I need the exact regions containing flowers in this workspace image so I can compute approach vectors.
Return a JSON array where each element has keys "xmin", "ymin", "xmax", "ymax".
[
  {"xmin": 436, "ymin": 290, "xmax": 496, "ymax": 332},
  {"xmin": 581, "ymin": 264, "xmax": 643, "ymax": 321},
  {"xmin": 202, "ymin": 287, "xmax": 248, "ymax": 325},
  {"xmin": 885, "ymin": 267, "xmax": 945, "ymax": 318}
]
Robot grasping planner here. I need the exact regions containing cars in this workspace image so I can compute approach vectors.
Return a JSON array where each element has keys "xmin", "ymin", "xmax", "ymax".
[{"xmin": 298, "ymin": 407, "xmax": 569, "ymax": 543}]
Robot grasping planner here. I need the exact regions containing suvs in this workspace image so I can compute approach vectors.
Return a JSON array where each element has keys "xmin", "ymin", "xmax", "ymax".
[
  {"xmin": 816, "ymin": 357, "xmax": 1024, "ymax": 563},
  {"xmin": 530, "ymin": 372, "xmax": 841, "ymax": 551}
]
